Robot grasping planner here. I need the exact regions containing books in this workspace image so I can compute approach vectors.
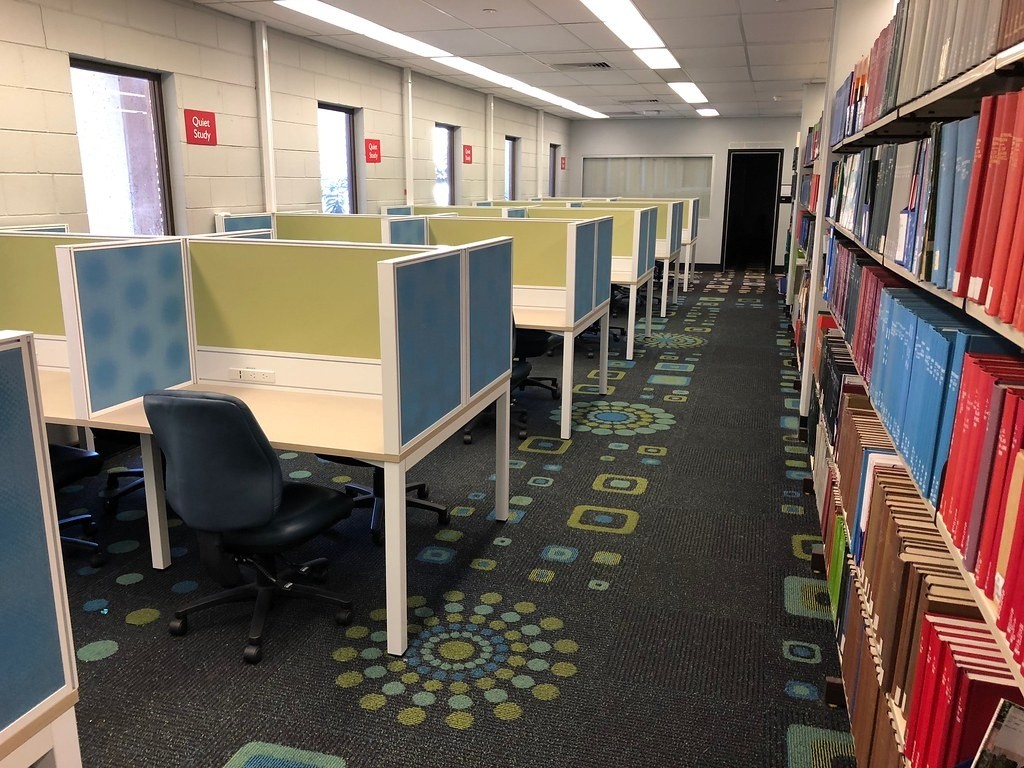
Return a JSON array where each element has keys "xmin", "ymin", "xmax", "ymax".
[{"xmin": 783, "ymin": 0, "xmax": 1024, "ymax": 768}]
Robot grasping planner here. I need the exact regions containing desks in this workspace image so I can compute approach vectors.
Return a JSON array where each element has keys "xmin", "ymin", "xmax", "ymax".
[{"xmin": 0, "ymin": 196, "xmax": 704, "ymax": 768}]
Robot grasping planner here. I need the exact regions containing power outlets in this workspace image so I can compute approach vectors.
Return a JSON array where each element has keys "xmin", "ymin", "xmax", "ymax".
[{"xmin": 230, "ymin": 368, "xmax": 277, "ymax": 384}]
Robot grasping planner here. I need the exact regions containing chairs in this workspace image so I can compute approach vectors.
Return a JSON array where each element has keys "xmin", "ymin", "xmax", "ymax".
[
  {"xmin": 40, "ymin": 440, "xmax": 148, "ymax": 566},
  {"xmin": 459, "ymin": 312, "xmax": 527, "ymax": 439},
  {"xmin": 507, "ymin": 325, "xmax": 558, "ymax": 399},
  {"xmin": 140, "ymin": 393, "xmax": 354, "ymax": 664}
]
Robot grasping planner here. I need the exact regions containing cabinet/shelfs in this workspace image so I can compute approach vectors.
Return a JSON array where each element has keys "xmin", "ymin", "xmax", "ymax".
[{"xmin": 787, "ymin": 43, "xmax": 1023, "ymax": 768}]
